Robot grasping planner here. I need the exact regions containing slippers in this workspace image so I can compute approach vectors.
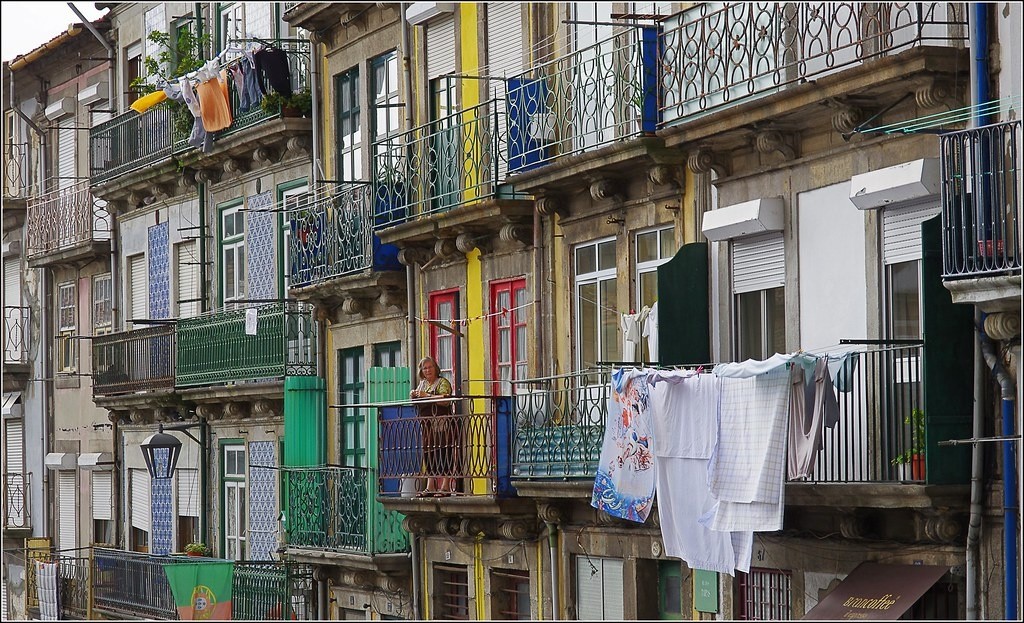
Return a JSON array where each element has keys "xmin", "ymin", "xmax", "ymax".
[
  {"xmin": 415, "ymin": 488, "xmax": 437, "ymax": 497},
  {"xmin": 434, "ymin": 488, "xmax": 451, "ymax": 497}
]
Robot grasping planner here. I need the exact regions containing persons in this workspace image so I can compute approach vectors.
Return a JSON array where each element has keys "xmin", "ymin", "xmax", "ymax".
[{"xmin": 410, "ymin": 356, "xmax": 458, "ymax": 498}]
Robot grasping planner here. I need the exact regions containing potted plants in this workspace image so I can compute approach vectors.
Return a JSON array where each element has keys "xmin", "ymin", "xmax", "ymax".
[
  {"xmin": 293, "ymin": 209, "xmax": 318, "ymax": 246},
  {"xmin": 184, "ymin": 543, "xmax": 211, "ymax": 558},
  {"xmin": 891, "ymin": 404, "xmax": 924, "ymax": 483},
  {"xmin": 617, "ymin": 73, "xmax": 643, "ymax": 130},
  {"xmin": 260, "ymin": 88, "xmax": 312, "ymax": 118}
]
